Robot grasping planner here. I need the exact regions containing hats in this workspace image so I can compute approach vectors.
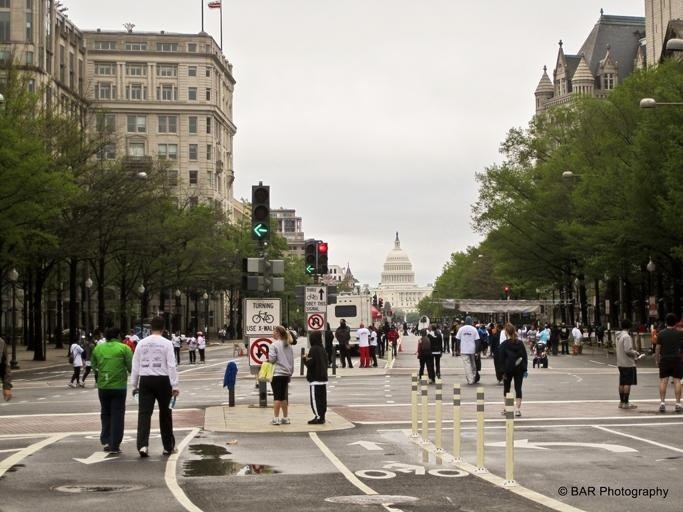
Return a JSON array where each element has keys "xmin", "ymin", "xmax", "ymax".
[{"xmin": 466, "ymin": 316, "xmax": 472, "ymax": 325}]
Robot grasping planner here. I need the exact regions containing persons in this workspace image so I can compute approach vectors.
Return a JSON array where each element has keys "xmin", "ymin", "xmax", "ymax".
[
  {"xmin": 0, "ymin": 337, "xmax": 13, "ymax": 402},
  {"xmin": 281, "ymin": 315, "xmax": 683, "ymax": 384},
  {"xmin": 217, "ymin": 325, "xmax": 234, "ymax": 342},
  {"xmin": 614, "ymin": 320, "xmax": 640, "ymax": 410},
  {"xmin": 65, "ymin": 327, "xmax": 205, "ymax": 389},
  {"xmin": 494, "ymin": 324, "xmax": 527, "ymax": 416},
  {"xmin": 130, "ymin": 317, "xmax": 179, "ymax": 457},
  {"xmin": 90, "ymin": 328, "xmax": 134, "ymax": 454},
  {"xmin": 304, "ymin": 331, "xmax": 328, "ymax": 424},
  {"xmin": 268, "ymin": 326, "xmax": 294, "ymax": 425},
  {"xmin": 655, "ymin": 313, "xmax": 682, "ymax": 412}
]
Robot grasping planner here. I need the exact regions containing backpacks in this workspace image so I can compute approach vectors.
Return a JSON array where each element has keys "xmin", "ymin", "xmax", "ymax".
[{"xmin": 68, "ymin": 352, "xmax": 75, "ymax": 364}]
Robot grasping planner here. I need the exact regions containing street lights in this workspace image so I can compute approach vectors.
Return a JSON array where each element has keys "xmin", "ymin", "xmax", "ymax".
[
  {"xmin": 664, "ymin": 34, "xmax": 682, "ymax": 53},
  {"xmin": 644, "ymin": 253, "xmax": 656, "ymax": 331},
  {"xmin": 203, "ymin": 293, "xmax": 212, "ymax": 343},
  {"xmin": 133, "ymin": 283, "xmax": 148, "ymax": 339},
  {"xmin": 8, "ymin": 262, "xmax": 20, "ymax": 371},
  {"xmin": 171, "ymin": 287, "xmax": 184, "ymax": 334},
  {"xmin": 637, "ymin": 94, "xmax": 682, "ymax": 109},
  {"xmin": 82, "ymin": 272, "xmax": 96, "ymax": 344},
  {"xmin": 561, "ymin": 169, "xmax": 626, "ymax": 323}
]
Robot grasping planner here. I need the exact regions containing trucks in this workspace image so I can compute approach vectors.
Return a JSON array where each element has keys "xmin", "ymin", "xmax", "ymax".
[{"xmin": 314, "ymin": 295, "xmax": 371, "ymax": 353}]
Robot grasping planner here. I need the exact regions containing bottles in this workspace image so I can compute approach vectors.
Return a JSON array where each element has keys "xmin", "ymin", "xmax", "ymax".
[{"xmin": 167, "ymin": 393, "xmax": 176, "ymax": 410}]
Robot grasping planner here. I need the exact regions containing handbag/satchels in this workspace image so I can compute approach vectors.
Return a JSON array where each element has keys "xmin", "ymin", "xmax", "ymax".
[{"xmin": 257, "ymin": 361, "xmax": 274, "ymax": 383}]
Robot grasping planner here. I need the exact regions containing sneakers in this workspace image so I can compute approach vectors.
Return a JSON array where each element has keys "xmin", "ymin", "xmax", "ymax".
[
  {"xmin": 109, "ymin": 447, "xmax": 121, "ymax": 454},
  {"xmin": 515, "ymin": 411, "xmax": 521, "ymax": 416},
  {"xmin": 617, "ymin": 402, "xmax": 623, "ymax": 408},
  {"xmin": 622, "ymin": 403, "xmax": 637, "ymax": 409},
  {"xmin": 103, "ymin": 444, "xmax": 109, "ymax": 451},
  {"xmin": 675, "ymin": 403, "xmax": 682, "ymax": 412},
  {"xmin": 139, "ymin": 446, "xmax": 150, "ymax": 458},
  {"xmin": 308, "ymin": 418, "xmax": 323, "ymax": 424},
  {"xmin": 280, "ymin": 418, "xmax": 289, "ymax": 424},
  {"xmin": 163, "ymin": 447, "xmax": 178, "ymax": 454},
  {"xmin": 270, "ymin": 417, "xmax": 280, "ymax": 425},
  {"xmin": 658, "ymin": 402, "xmax": 666, "ymax": 411}
]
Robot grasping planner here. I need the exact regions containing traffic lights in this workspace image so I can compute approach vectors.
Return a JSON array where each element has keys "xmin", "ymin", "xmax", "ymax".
[
  {"xmin": 304, "ymin": 241, "xmax": 316, "ymax": 274},
  {"xmin": 251, "ymin": 184, "xmax": 270, "ymax": 239},
  {"xmin": 317, "ymin": 241, "xmax": 330, "ymax": 274},
  {"xmin": 504, "ymin": 285, "xmax": 512, "ymax": 300}
]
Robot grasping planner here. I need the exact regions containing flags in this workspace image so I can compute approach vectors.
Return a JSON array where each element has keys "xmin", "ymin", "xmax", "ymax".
[{"xmin": 208, "ymin": 0, "xmax": 220, "ymax": 9}]
